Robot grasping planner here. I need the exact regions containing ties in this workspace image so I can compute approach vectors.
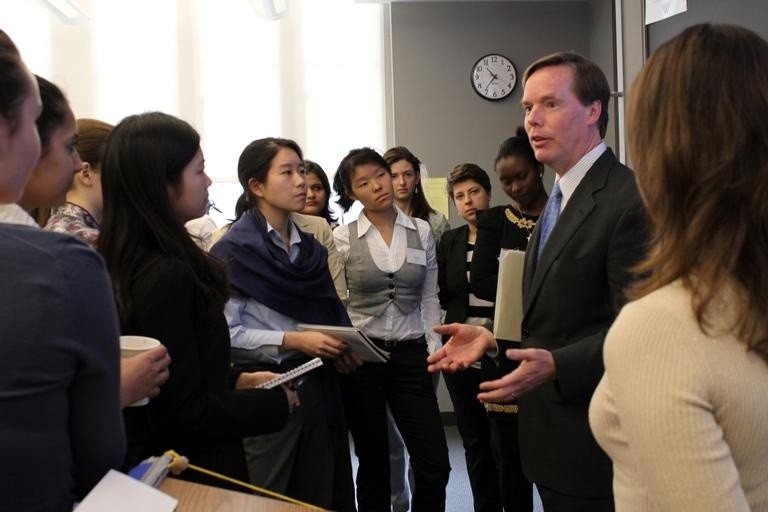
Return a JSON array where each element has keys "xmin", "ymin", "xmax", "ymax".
[{"xmin": 537, "ymin": 181, "xmax": 562, "ymax": 267}]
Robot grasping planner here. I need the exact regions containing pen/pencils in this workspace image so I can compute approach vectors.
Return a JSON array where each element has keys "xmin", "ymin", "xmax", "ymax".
[{"xmin": 290, "ymin": 373, "xmax": 309, "ymax": 392}]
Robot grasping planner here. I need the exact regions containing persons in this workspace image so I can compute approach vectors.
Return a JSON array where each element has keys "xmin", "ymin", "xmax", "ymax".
[
  {"xmin": 428, "ymin": 53, "xmax": 656, "ymax": 511},
  {"xmin": 0, "ymin": 30, "xmax": 549, "ymax": 511},
  {"xmin": 589, "ymin": 24, "xmax": 768, "ymax": 511}
]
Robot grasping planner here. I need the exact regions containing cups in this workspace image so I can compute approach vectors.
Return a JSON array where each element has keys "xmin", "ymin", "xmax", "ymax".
[{"xmin": 117, "ymin": 334, "xmax": 161, "ymax": 407}]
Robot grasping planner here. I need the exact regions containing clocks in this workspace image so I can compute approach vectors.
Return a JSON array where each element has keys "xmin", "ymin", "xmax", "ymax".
[{"xmin": 470, "ymin": 54, "xmax": 518, "ymax": 101}]
